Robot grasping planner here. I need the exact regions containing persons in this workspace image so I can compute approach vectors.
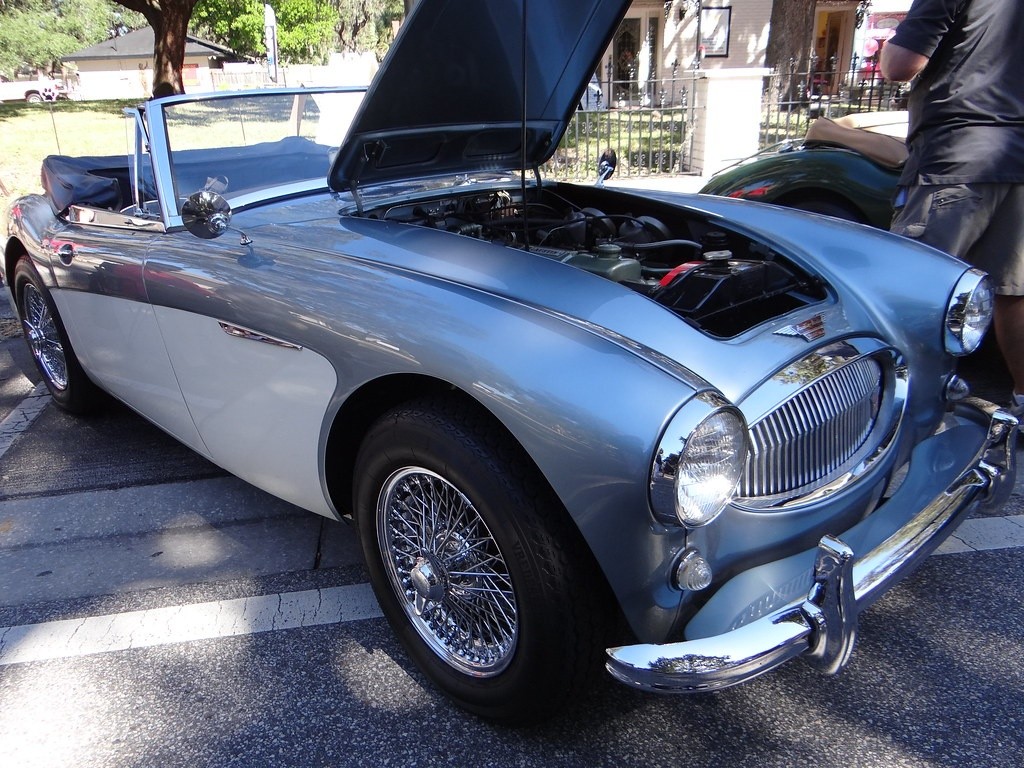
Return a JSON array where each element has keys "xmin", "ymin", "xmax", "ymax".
[{"xmin": 880, "ymin": 0, "xmax": 1024, "ymax": 435}]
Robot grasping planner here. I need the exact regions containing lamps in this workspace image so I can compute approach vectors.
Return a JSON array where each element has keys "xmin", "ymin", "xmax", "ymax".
[{"xmin": 679, "ymin": 0, "xmax": 691, "ymax": 20}]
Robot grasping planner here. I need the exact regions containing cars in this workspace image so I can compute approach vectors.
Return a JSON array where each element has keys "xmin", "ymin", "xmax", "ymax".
[
  {"xmin": 0, "ymin": 73, "xmax": 66, "ymax": 104},
  {"xmin": 576, "ymin": 83, "xmax": 602, "ymax": 110},
  {"xmin": 698, "ymin": 138, "xmax": 1018, "ymax": 404},
  {"xmin": 0, "ymin": 0, "xmax": 1023, "ymax": 727}
]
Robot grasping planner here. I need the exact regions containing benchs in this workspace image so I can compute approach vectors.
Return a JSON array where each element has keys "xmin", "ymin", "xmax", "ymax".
[
  {"xmin": 844, "ymin": 85, "xmax": 896, "ymax": 105},
  {"xmin": 42, "ymin": 132, "xmax": 345, "ymax": 210}
]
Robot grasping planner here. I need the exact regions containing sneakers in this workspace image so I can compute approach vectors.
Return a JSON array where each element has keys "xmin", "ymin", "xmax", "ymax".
[{"xmin": 1004, "ymin": 390, "xmax": 1024, "ymax": 434}]
